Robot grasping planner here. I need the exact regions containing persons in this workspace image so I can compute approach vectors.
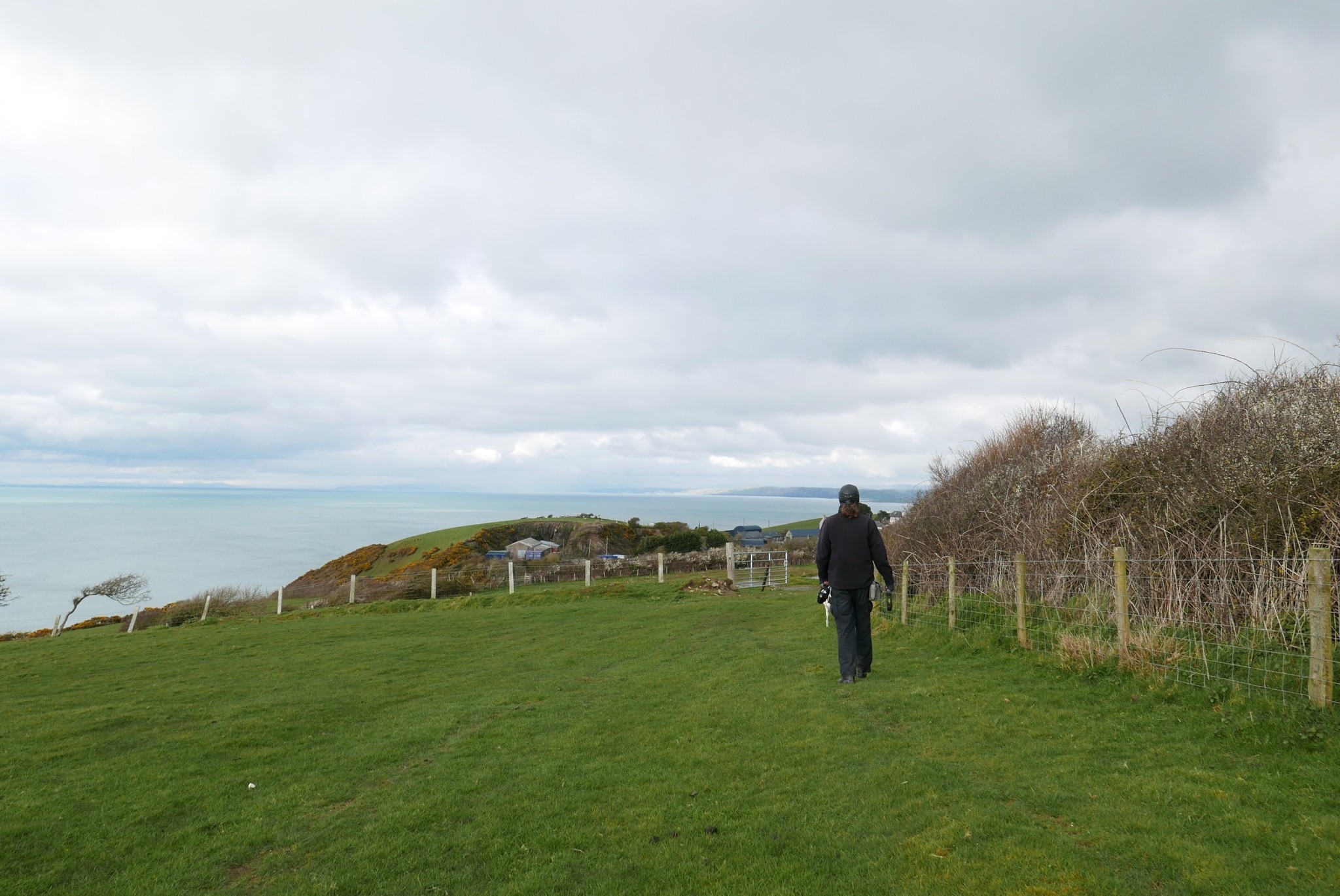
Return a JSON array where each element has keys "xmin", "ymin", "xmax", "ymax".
[{"xmin": 815, "ymin": 484, "xmax": 895, "ymax": 684}]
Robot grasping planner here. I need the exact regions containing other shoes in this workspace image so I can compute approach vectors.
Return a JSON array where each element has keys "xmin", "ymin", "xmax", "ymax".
[
  {"xmin": 857, "ymin": 668, "xmax": 867, "ymax": 678},
  {"xmin": 839, "ymin": 675, "xmax": 855, "ymax": 684}
]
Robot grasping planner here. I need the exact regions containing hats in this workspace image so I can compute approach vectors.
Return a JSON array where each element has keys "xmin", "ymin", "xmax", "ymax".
[{"xmin": 838, "ymin": 484, "xmax": 859, "ymax": 505}]
